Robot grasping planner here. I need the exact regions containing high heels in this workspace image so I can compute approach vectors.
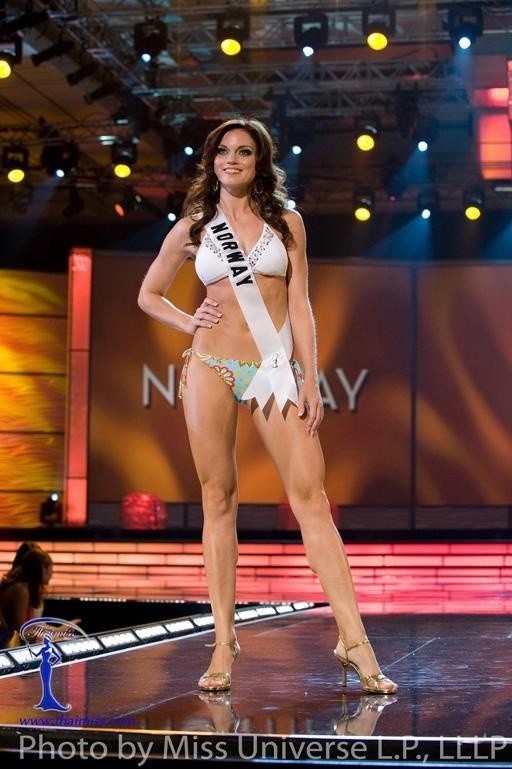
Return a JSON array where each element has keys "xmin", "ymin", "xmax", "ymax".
[
  {"xmin": 332, "ymin": 635, "xmax": 398, "ymax": 693},
  {"xmin": 198, "ymin": 639, "xmax": 240, "ymax": 690}
]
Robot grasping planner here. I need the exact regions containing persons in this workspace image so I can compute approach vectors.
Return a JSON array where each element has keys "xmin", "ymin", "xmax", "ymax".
[
  {"xmin": 1, "ymin": 548, "xmax": 69, "ymax": 650},
  {"xmin": 139, "ymin": 117, "xmax": 398, "ymax": 696},
  {"xmin": 200, "ymin": 697, "xmax": 397, "ymax": 736},
  {"xmin": 1, "ymin": 542, "xmax": 83, "ymax": 635}
]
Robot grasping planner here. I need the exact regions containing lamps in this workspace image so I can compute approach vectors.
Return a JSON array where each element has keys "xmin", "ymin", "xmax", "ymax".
[
  {"xmin": 0, "ymin": 8, "xmax": 489, "ymax": 87},
  {"xmin": 0, "ymin": 110, "xmax": 489, "ymax": 225}
]
